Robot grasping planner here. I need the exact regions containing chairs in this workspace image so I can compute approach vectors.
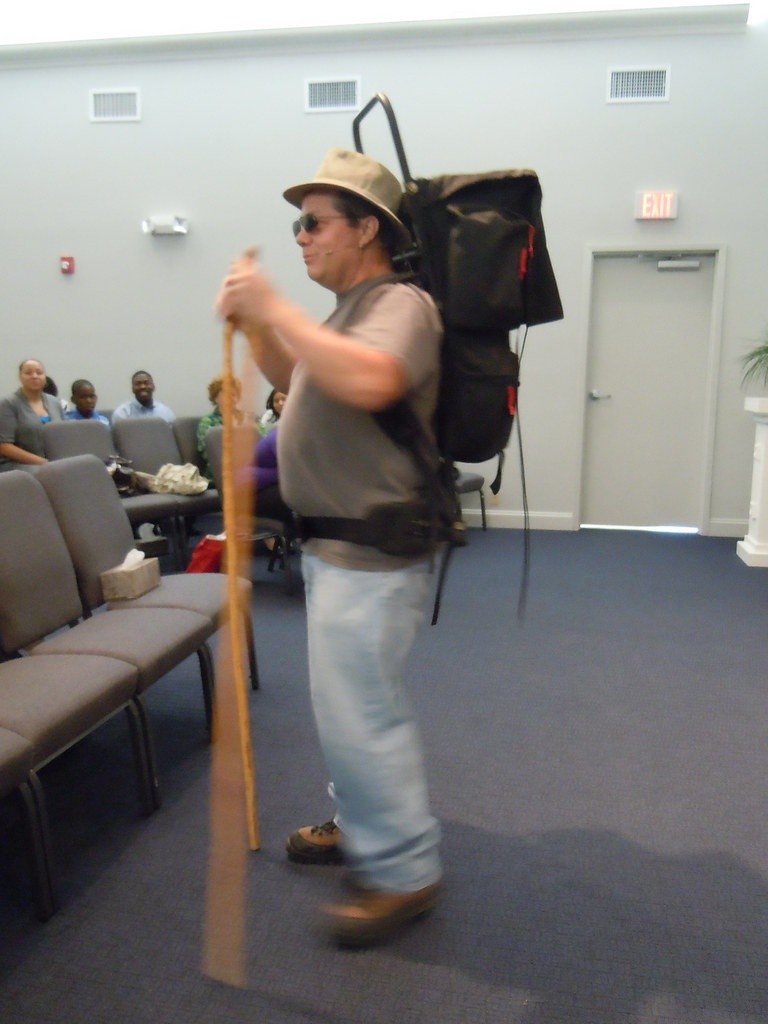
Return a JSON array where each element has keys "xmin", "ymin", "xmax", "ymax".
[
  {"xmin": 0, "ymin": 415, "xmax": 293, "ymax": 921},
  {"xmin": 455, "ymin": 472, "xmax": 489, "ymax": 543}
]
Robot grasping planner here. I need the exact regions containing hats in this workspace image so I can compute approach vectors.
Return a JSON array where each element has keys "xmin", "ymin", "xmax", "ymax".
[{"xmin": 282, "ymin": 148, "xmax": 413, "ymax": 254}]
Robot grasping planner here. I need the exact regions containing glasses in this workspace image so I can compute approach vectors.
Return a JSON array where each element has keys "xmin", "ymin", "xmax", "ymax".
[{"xmin": 292, "ymin": 212, "xmax": 360, "ymax": 236}]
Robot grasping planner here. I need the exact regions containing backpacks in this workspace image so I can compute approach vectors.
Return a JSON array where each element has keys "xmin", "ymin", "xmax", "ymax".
[{"xmin": 399, "ymin": 168, "xmax": 565, "ymax": 464}]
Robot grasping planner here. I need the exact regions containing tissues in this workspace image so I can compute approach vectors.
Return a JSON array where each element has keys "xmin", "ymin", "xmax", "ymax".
[{"xmin": 100, "ymin": 548, "xmax": 162, "ymax": 602}]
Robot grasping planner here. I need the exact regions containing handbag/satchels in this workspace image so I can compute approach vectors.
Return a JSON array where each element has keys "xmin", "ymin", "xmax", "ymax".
[{"xmin": 185, "ymin": 530, "xmax": 227, "ymax": 573}]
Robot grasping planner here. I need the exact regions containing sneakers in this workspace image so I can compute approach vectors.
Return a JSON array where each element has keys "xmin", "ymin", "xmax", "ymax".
[
  {"xmin": 285, "ymin": 820, "xmax": 344, "ymax": 864},
  {"xmin": 313, "ymin": 872, "xmax": 445, "ymax": 943}
]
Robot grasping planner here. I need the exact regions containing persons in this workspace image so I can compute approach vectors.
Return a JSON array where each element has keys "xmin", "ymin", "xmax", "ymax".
[{"xmin": 0, "ymin": 147, "xmax": 470, "ymax": 934}]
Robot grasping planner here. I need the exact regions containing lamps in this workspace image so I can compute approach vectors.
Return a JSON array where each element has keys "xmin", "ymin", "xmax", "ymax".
[{"xmin": 141, "ymin": 214, "xmax": 187, "ymax": 236}]
